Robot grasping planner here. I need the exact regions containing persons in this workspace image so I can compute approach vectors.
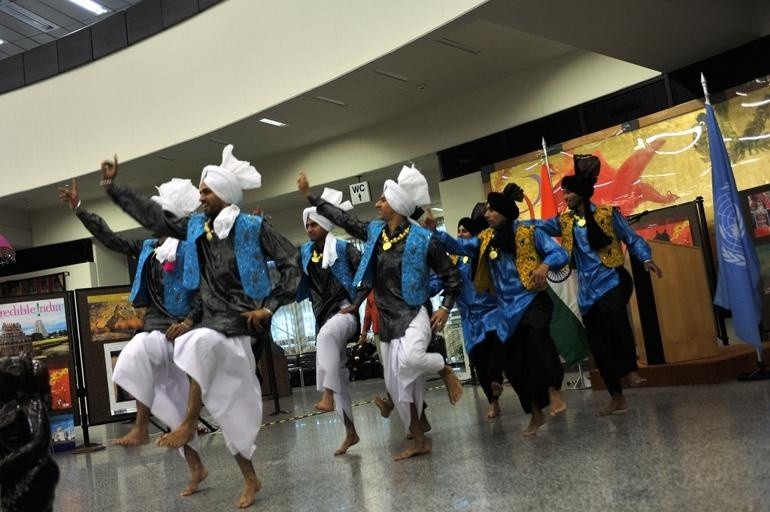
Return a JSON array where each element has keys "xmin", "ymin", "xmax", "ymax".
[
  {"xmin": 58, "ymin": 178, "xmax": 209, "ymax": 496},
  {"xmin": 100, "ymin": 144, "xmax": 303, "ymax": 508}
]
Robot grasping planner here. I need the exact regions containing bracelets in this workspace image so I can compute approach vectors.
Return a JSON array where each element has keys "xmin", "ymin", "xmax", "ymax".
[
  {"xmin": 74, "ymin": 200, "xmax": 81, "ymax": 211},
  {"xmin": 98, "ymin": 179, "xmax": 113, "ymax": 187}
]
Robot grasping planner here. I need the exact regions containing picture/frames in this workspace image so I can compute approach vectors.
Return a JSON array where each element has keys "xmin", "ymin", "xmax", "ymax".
[
  {"xmin": 740, "ymin": 185, "xmax": 770, "ymax": 246},
  {"xmin": 102, "ymin": 340, "xmax": 138, "ymax": 417},
  {"xmin": 627, "ymin": 199, "xmax": 702, "ymax": 254}
]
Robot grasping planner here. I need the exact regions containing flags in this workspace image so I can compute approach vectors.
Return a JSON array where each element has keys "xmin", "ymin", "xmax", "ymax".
[{"xmin": 705, "ymin": 102, "xmax": 764, "ymax": 352}]
